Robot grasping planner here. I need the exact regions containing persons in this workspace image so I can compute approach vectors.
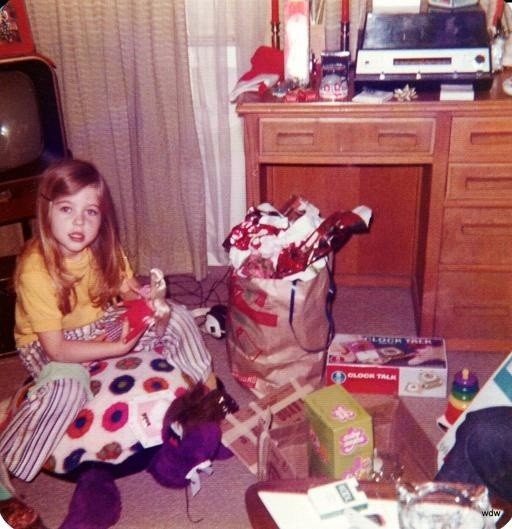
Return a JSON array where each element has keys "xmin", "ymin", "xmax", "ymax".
[
  {"xmin": 94, "ymin": 292, "xmax": 173, "ymax": 346},
  {"xmin": 1, "ymin": 158, "xmax": 237, "ymax": 529}
]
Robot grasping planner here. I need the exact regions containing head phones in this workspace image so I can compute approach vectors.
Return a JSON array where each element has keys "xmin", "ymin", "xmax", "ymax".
[{"xmin": 187, "ymin": 300, "xmax": 231, "ymax": 339}]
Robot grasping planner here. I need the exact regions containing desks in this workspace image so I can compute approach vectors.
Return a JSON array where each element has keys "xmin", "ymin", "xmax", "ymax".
[
  {"xmin": 0, "ymin": 197, "xmax": 56, "ymax": 356},
  {"xmin": 245, "ymin": 476, "xmax": 512, "ymax": 529}
]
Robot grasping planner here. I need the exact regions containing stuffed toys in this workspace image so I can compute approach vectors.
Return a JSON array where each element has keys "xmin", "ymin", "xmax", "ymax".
[{"xmin": 9, "ymin": 350, "xmax": 236, "ymax": 529}]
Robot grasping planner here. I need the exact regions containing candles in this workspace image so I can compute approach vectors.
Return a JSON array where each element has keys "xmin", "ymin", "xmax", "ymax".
[
  {"xmin": 271, "ymin": 0, "xmax": 279, "ymax": 24},
  {"xmin": 341, "ymin": 0, "xmax": 349, "ymax": 24}
]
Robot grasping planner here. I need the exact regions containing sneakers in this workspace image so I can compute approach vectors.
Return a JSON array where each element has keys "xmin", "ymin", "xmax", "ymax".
[{"xmin": 207, "ymin": 376, "xmax": 240, "ymax": 420}]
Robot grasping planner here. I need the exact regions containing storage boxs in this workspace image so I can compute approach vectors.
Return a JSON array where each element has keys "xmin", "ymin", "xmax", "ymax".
[{"xmin": 221, "ymin": 330, "xmax": 449, "ymax": 483}]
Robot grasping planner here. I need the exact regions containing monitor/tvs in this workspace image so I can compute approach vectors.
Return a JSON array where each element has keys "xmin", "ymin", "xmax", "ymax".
[{"xmin": 0, "ymin": 56, "xmax": 72, "ymax": 227}]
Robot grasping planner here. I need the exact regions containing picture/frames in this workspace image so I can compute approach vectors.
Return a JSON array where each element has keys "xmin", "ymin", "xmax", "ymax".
[{"xmin": 0, "ymin": 0, "xmax": 36, "ymax": 57}]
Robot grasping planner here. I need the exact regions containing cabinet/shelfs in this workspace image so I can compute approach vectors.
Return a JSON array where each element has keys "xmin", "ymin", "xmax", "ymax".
[{"xmin": 235, "ymin": 70, "xmax": 512, "ymax": 353}]
